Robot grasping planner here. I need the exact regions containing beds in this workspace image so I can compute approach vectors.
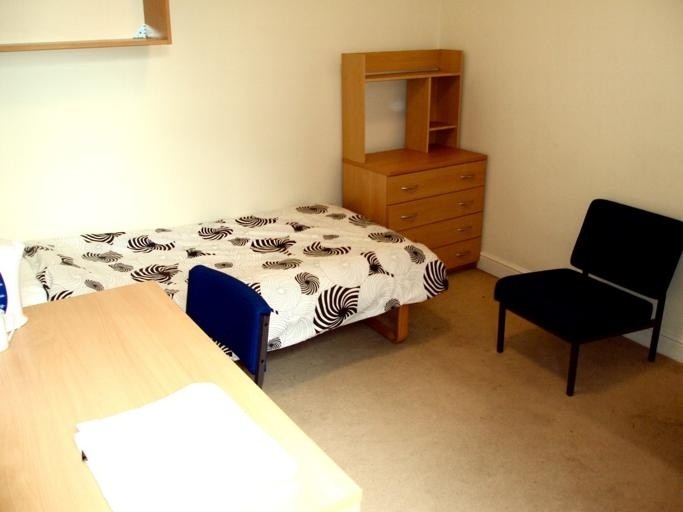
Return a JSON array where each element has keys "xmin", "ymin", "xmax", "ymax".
[{"xmin": 0, "ymin": 202, "xmax": 451, "ymax": 362}]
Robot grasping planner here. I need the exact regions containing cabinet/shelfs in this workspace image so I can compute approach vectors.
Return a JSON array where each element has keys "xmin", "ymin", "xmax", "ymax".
[{"xmin": 340, "ymin": 49, "xmax": 488, "ymax": 274}]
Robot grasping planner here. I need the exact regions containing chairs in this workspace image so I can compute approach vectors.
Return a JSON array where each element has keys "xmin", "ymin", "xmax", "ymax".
[
  {"xmin": 186, "ymin": 265, "xmax": 272, "ymax": 389},
  {"xmin": 493, "ymin": 198, "xmax": 683, "ymax": 397}
]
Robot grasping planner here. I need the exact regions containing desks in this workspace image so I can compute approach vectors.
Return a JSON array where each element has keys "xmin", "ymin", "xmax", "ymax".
[{"xmin": 0, "ymin": 280, "xmax": 365, "ymax": 512}]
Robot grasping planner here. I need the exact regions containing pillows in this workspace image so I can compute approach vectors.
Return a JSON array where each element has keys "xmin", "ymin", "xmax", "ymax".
[{"xmin": 0, "ymin": 241, "xmax": 46, "ymax": 304}]
